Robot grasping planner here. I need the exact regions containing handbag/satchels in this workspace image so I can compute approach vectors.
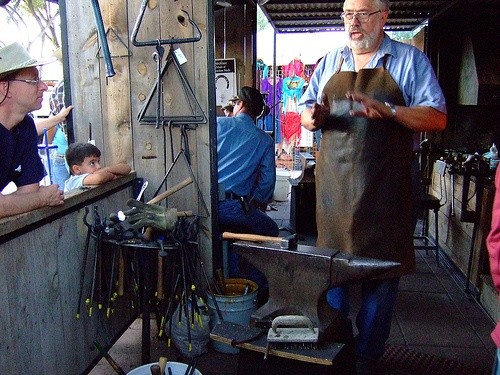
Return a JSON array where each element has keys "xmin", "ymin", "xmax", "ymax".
[{"xmin": 170, "ymin": 291, "xmax": 212, "ymax": 358}]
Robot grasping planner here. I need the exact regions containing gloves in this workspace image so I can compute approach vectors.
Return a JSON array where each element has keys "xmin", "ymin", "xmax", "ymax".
[{"xmin": 108, "ymin": 198, "xmax": 178, "ymax": 232}]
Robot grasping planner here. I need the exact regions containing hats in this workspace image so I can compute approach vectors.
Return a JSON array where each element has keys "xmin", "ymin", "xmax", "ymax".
[
  {"xmin": 222, "ymin": 105, "xmax": 233, "ymax": 112},
  {"xmin": 0, "ymin": 41, "xmax": 45, "ymax": 75},
  {"xmin": 229, "ymin": 86, "xmax": 264, "ymax": 116}
]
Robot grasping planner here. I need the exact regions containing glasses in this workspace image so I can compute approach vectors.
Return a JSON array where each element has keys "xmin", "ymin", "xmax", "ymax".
[
  {"xmin": 232, "ymin": 101, "xmax": 241, "ymax": 105},
  {"xmin": 12, "ymin": 76, "xmax": 39, "ymax": 83},
  {"xmin": 340, "ymin": 10, "xmax": 381, "ymax": 24}
]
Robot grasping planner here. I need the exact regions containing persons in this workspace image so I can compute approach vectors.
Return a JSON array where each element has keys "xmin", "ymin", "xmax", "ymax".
[
  {"xmin": 218, "ymin": 85, "xmax": 279, "ymax": 237},
  {"xmin": 487, "ymin": 162, "xmax": 500, "ymax": 375},
  {"xmin": 0, "ymin": 41, "xmax": 64, "ymax": 218},
  {"xmin": 296, "ymin": 0, "xmax": 447, "ymax": 375},
  {"xmin": 65, "ymin": 141, "xmax": 132, "ymax": 195},
  {"xmin": 27, "ymin": 105, "xmax": 74, "ymax": 136},
  {"xmin": 276, "ymin": 57, "xmax": 321, "ymax": 170}
]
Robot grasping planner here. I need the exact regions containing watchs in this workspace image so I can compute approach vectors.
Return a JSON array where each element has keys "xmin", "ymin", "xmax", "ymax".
[{"xmin": 384, "ymin": 101, "xmax": 397, "ymax": 120}]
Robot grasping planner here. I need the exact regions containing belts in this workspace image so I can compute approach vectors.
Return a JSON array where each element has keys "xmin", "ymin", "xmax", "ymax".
[{"xmin": 225, "ymin": 193, "xmax": 247, "ymax": 200}]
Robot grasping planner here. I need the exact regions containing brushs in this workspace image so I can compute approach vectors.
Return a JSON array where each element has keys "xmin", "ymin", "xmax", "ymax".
[{"xmin": 266, "ymin": 314, "xmax": 319, "ymax": 350}]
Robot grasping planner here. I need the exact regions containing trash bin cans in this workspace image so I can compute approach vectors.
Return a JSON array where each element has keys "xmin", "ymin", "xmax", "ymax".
[{"xmin": 206, "ymin": 279, "xmax": 259, "ymax": 355}]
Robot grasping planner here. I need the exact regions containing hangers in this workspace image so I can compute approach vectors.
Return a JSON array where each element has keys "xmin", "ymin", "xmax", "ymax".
[
  {"xmin": 269, "ymin": 67, "xmax": 280, "ymax": 80},
  {"xmin": 291, "ymin": 70, "xmax": 308, "ymax": 86}
]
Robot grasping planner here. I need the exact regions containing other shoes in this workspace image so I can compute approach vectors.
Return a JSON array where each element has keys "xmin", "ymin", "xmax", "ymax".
[
  {"xmin": 353, "ymin": 333, "xmax": 380, "ymax": 375},
  {"xmin": 321, "ymin": 301, "xmax": 349, "ymax": 321}
]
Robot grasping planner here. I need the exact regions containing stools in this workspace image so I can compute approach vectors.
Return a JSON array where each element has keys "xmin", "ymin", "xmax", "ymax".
[
  {"xmin": 413, "ymin": 195, "xmax": 441, "ymax": 266},
  {"xmin": 93, "ymin": 238, "xmax": 191, "ymax": 375}
]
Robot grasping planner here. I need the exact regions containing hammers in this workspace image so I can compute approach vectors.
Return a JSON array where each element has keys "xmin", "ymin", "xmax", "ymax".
[{"xmin": 221, "ymin": 231, "xmax": 299, "ymax": 249}]
